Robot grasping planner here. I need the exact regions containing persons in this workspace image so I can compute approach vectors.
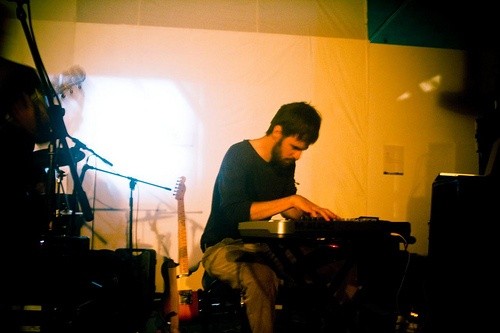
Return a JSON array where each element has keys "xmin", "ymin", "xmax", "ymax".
[
  {"xmin": 200, "ymin": 102, "xmax": 361, "ymax": 333},
  {"xmin": 0, "ymin": 56, "xmax": 52, "ymax": 333}
]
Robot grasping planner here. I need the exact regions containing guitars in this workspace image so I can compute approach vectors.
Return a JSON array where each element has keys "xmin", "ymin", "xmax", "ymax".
[{"xmin": 173, "ymin": 176, "xmax": 198, "ymax": 319}]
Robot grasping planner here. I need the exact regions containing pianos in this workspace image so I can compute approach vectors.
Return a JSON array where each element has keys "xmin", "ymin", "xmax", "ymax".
[{"xmin": 238, "ymin": 212, "xmax": 411, "ymax": 246}]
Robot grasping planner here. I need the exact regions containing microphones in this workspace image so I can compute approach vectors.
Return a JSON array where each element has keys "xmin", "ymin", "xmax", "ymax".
[{"xmin": 79, "ymin": 158, "xmax": 89, "ymax": 185}]
